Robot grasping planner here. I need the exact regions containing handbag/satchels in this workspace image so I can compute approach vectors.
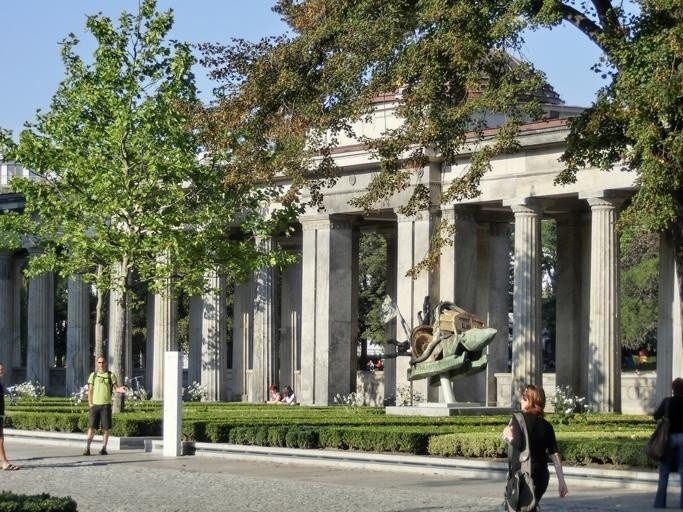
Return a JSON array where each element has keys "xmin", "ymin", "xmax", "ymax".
[
  {"xmin": 502, "ymin": 451, "xmax": 536, "ymax": 512},
  {"xmin": 645, "ymin": 416, "xmax": 669, "ymax": 462}
]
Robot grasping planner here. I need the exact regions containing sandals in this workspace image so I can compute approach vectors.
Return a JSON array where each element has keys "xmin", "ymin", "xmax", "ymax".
[{"xmin": 5, "ymin": 464, "xmax": 19, "ymax": 470}]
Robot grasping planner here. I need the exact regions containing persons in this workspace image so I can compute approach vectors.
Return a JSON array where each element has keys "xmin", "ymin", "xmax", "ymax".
[
  {"xmin": 81, "ymin": 355, "xmax": 126, "ymax": 454},
  {"xmin": 651, "ymin": 377, "xmax": 683, "ymax": 510},
  {"xmin": 375, "ymin": 360, "xmax": 384, "ymax": 371},
  {"xmin": 280, "ymin": 384, "xmax": 296, "ymax": 406},
  {"xmin": 367, "ymin": 360, "xmax": 375, "ymax": 371},
  {"xmin": 0, "ymin": 362, "xmax": 20, "ymax": 471},
  {"xmin": 501, "ymin": 383, "xmax": 568, "ymax": 512},
  {"xmin": 266, "ymin": 385, "xmax": 282, "ymax": 404}
]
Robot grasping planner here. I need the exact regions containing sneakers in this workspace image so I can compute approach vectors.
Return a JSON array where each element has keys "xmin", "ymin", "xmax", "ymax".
[
  {"xmin": 83, "ymin": 451, "xmax": 90, "ymax": 455},
  {"xmin": 99, "ymin": 450, "xmax": 107, "ymax": 455}
]
